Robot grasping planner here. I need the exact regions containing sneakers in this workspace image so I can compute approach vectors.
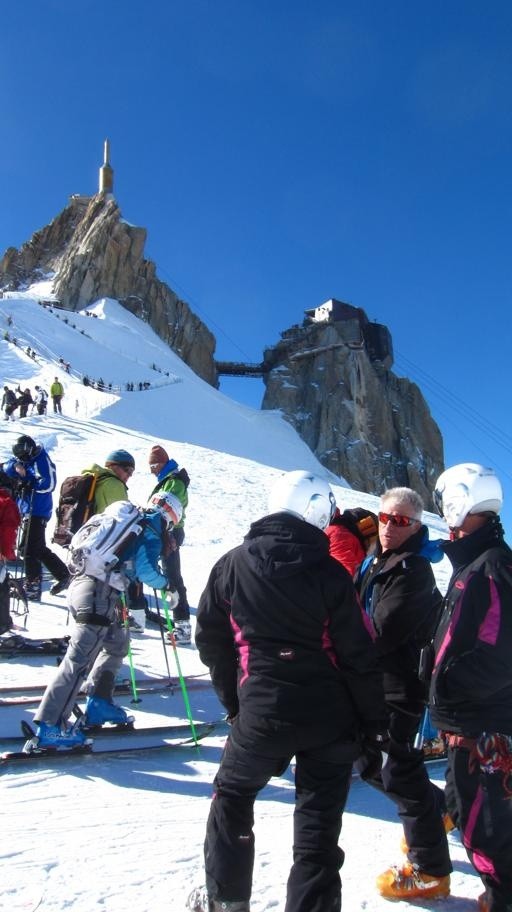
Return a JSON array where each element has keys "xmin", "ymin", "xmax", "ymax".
[
  {"xmin": 402, "ymin": 813, "xmax": 456, "ymax": 854},
  {"xmin": 166, "ymin": 621, "xmax": 191, "ymax": 645},
  {"xmin": 120, "ymin": 610, "xmax": 147, "ymax": 633},
  {"xmin": 85, "ymin": 695, "xmax": 127, "ymax": 724},
  {"xmin": 36, "ymin": 720, "xmax": 84, "ymax": 749},
  {"xmin": 376, "ymin": 862, "xmax": 450, "ymax": 903},
  {"xmin": 186, "ymin": 885, "xmax": 250, "ymax": 912},
  {"xmin": 9, "ymin": 579, "xmax": 41, "ymax": 602},
  {"xmin": 50, "ymin": 571, "xmax": 72, "ymax": 595},
  {"xmin": 0, "ymin": 630, "xmax": 24, "ymax": 648},
  {"xmin": 419, "ymin": 733, "xmax": 447, "ymax": 762}
]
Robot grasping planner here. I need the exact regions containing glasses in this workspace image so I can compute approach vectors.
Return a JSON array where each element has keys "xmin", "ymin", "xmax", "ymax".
[
  {"xmin": 149, "ymin": 463, "xmax": 158, "ymax": 468},
  {"xmin": 122, "ymin": 465, "xmax": 134, "ymax": 473},
  {"xmin": 378, "ymin": 513, "xmax": 420, "ymax": 528}
]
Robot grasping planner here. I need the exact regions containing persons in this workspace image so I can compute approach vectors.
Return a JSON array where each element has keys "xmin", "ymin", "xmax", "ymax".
[
  {"xmin": 2, "ymin": 435, "xmax": 70, "ymax": 600},
  {"xmin": 83, "ymin": 374, "xmax": 170, "ymax": 392},
  {"xmin": 0, "ymin": 486, "xmax": 22, "ymax": 635},
  {"xmin": 187, "ymin": 468, "xmax": 365, "ymax": 912},
  {"xmin": 80, "ymin": 449, "xmax": 136, "ymax": 515},
  {"xmin": 32, "ymin": 491, "xmax": 184, "ymax": 747},
  {"xmin": 353, "ymin": 486, "xmax": 458, "ymax": 900},
  {"xmin": 4, "ymin": 314, "xmax": 76, "ymax": 374},
  {"xmin": 146, "ymin": 445, "xmax": 192, "ymax": 646},
  {"xmin": 416, "ymin": 461, "xmax": 512, "ymax": 912},
  {"xmin": 51, "ymin": 376, "xmax": 65, "ymax": 413},
  {"xmin": 1, "ymin": 386, "xmax": 17, "ymax": 422},
  {"xmin": 328, "ymin": 507, "xmax": 380, "ymax": 577},
  {"xmin": 17, "ymin": 383, "xmax": 36, "ymax": 417},
  {"xmin": 34, "ymin": 385, "xmax": 49, "ymax": 415}
]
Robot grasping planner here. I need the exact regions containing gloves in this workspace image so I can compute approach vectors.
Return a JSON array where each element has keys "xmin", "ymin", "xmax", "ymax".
[{"xmin": 163, "ymin": 583, "xmax": 179, "ymax": 608}]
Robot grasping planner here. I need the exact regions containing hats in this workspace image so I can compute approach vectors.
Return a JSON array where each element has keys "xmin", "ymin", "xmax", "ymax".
[
  {"xmin": 105, "ymin": 449, "xmax": 135, "ymax": 466},
  {"xmin": 149, "ymin": 446, "xmax": 169, "ymax": 464}
]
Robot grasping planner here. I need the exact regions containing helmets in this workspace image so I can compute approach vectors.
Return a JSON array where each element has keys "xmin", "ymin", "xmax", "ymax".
[
  {"xmin": 433, "ymin": 463, "xmax": 503, "ymax": 533},
  {"xmin": 269, "ymin": 471, "xmax": 335, "ymax": 529},
  {"xmin": 148, "ymin": 492, "xmax": 183, "ymax": 531},
  {"xmin": 12, "ymin": 435, "xmax": 35, "ymax": 462}
]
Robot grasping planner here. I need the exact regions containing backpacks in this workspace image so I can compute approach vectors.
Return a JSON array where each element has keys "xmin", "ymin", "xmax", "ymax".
[
  {"xmin": 52, "ymin": 474, "xmax": 128, "ymax": 550},
  {"xmin": 68, "ymin": 499, "xmax": 145, "ymax": 576},
  {"xmin": 336, "ymin": 507, "xmax": 379, "ymax": 553}
]
